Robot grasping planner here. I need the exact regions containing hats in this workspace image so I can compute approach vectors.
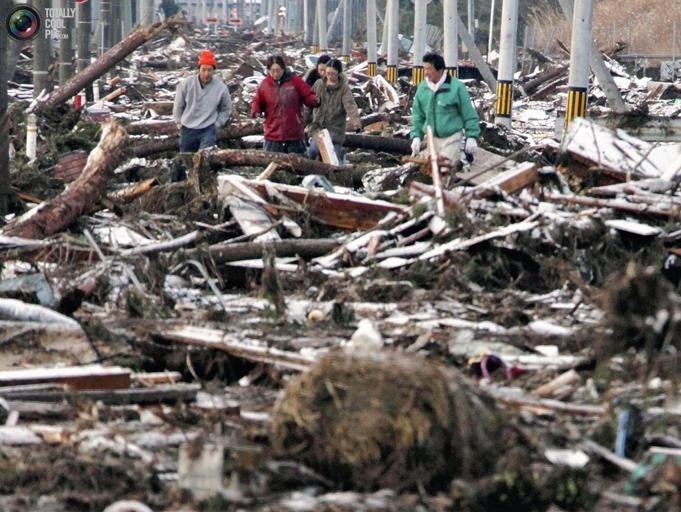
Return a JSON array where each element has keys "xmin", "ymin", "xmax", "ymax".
[{"xmin": 198, "ymin": 50, "xmax": 218, "ymax": 70}]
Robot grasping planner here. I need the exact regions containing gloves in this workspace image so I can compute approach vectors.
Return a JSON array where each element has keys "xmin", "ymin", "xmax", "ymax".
[
  {"xmin": 410, "ymin": 136, "xmax": 422, "ymax": 158},
  {"xmin": 464, "ymin": 136, "xmax": 479, "ymax": 154}
]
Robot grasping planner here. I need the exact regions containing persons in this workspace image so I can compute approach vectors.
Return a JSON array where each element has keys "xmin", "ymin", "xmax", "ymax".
[
  {"xmin": 301, "ymin": 59, "xmax": 363, "ymax": 164},
  {"xmin": 250, "ymin": 55, "xmax": 323, "ymax": 156},
  {"xmin": 409, "ymin": 53, "xmax": 482, "ymax": 177},
  {"xmin": 304, "ymin": 56, "xmax": 333, "ymax": 86},
  {"xmin": 172, "ymin": 50, "xmax": 233, "ymax": 155}
]
